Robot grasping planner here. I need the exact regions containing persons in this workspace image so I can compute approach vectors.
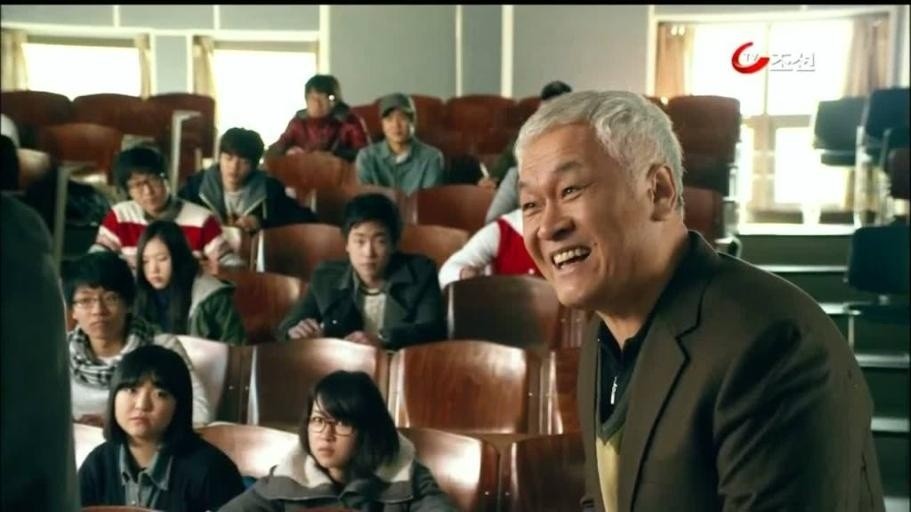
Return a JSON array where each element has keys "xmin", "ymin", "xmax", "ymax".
[
  {"xmin": 63, "ymin": 74, "xmax": 574, "ymax": 511},
  {"xmin": 512, "ymin": 87, "xmax": 889, "ymax": 512}
]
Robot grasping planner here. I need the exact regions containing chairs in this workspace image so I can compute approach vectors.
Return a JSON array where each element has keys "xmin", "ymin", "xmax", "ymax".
[{"xmin": 813, "ymin": 87, "xmax": 911, "ymax": 352}]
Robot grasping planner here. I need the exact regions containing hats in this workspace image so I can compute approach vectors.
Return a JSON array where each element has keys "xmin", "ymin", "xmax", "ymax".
[{"xmin": 378, "ymin": 93, "xmax": 418, "ymax": 118}]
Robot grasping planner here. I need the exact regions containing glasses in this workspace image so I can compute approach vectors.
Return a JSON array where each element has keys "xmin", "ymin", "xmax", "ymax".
[
  {"xmin": 125, "ymin": 174, "xmax": 166, "ymax": 192},
  {"xmin": 305, "ymin": 417, "xmax": 354, "ymax": 437},
  {"xmin": 71, "ymin": 294, "xmax": 122, "ymax": 309}
]
